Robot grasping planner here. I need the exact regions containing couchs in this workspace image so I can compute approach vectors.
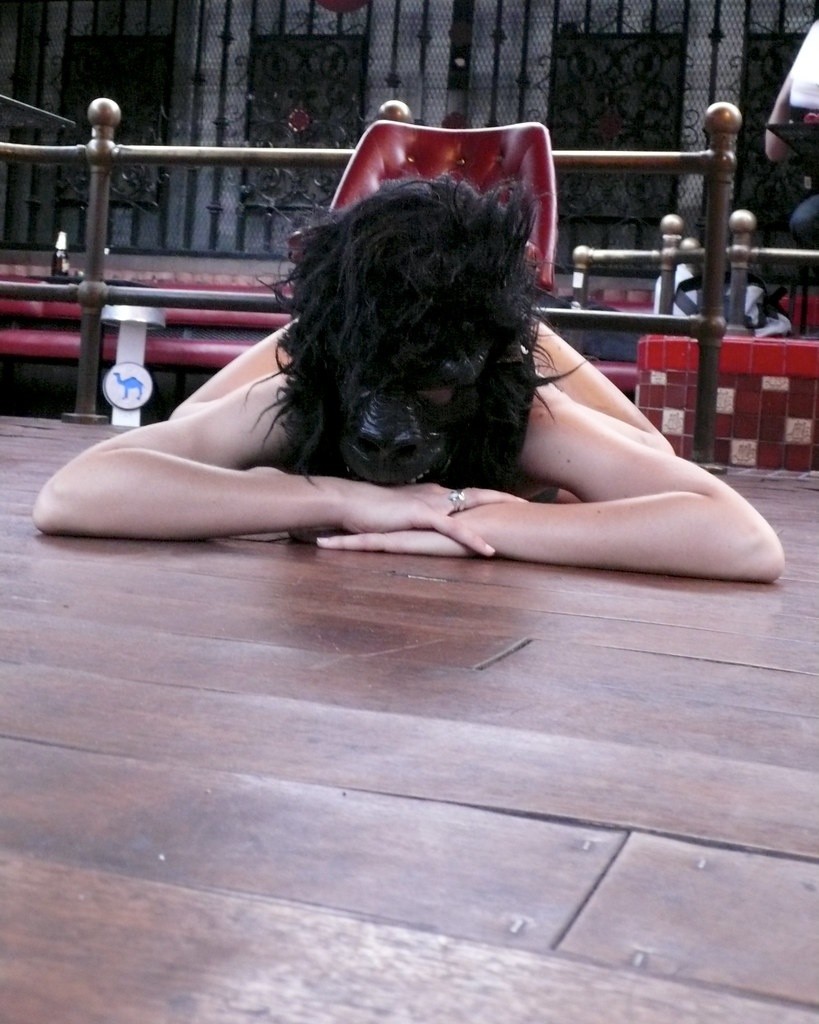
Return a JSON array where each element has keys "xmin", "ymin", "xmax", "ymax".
[{"xmin": 0, "ymin": 275, "xmax": 819, "ymax": 415}]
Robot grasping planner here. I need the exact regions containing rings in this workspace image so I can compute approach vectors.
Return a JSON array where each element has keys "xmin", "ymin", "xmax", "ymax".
[{"xmin": 450, "ymin": 486, "xmax": 467, "ymax": 512}]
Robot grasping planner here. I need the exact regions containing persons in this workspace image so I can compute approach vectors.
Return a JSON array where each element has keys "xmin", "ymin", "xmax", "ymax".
[{"xmin": 32, "ymin": 16, "xmax": 819, "ymax": 583}]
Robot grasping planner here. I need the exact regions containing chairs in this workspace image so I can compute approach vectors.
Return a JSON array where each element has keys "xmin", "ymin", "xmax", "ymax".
[{"xmin": 290, "ymin": 120, "xmax": 558, "ymax": 291}]
